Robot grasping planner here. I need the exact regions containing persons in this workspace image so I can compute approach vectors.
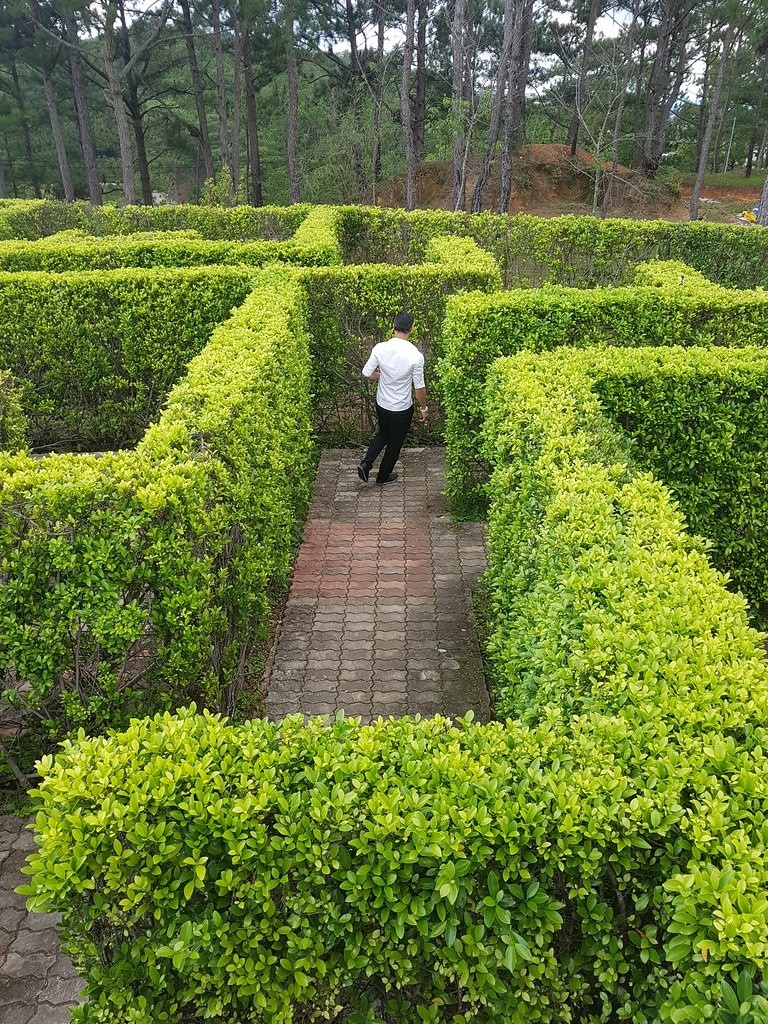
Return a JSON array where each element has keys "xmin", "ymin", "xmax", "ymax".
[
  {"xmin": 741, "ymin": 208, "xmax": 759, "ymax": 223},
  {"xmin": 357, "ymin": 311, "xmax": 428, "ymax": 484}
]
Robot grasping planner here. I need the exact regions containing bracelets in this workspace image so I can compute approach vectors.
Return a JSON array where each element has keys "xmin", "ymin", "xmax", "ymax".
[{"xmin": 419, "ymin": 405, "xmax": 428, "ymax": 412}]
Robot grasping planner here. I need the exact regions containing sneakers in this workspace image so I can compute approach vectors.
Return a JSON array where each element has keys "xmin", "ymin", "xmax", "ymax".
[
  {"xmin": 357, "ymin": 461, "xmax": 370, "ymax": 482},
  {"xmin": 376, "ymin": 473, "xmax": 399, "ymax": 486}
]
